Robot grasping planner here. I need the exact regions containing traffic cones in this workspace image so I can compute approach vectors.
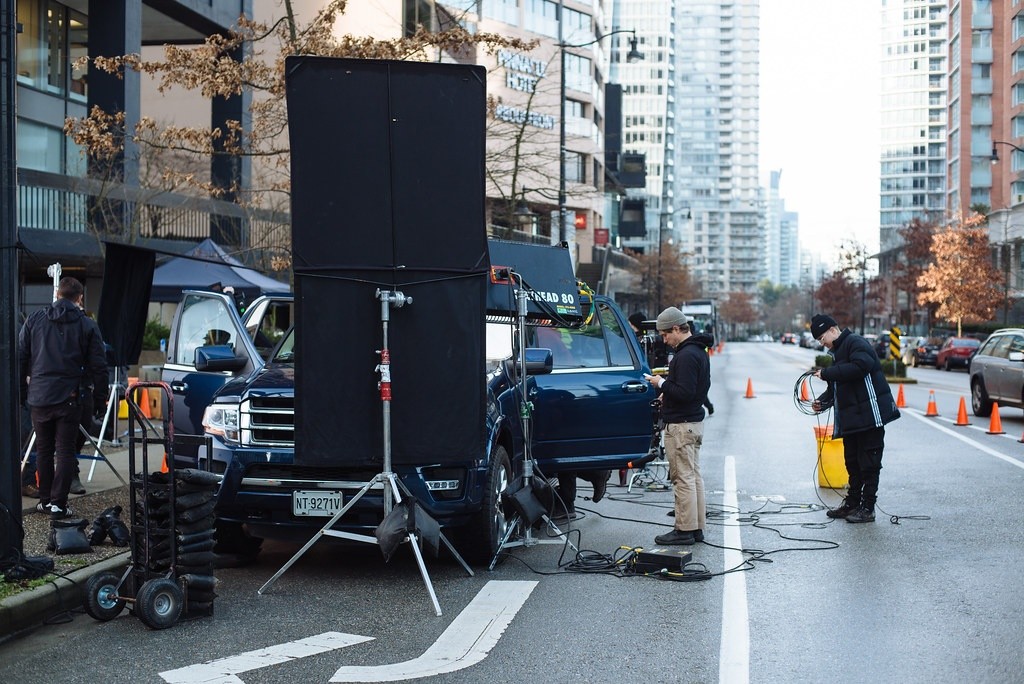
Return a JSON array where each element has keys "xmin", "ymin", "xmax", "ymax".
[
  {"xmin": 138, "ymin": 387, "xmax": 153, "ymax": 420},
  {"xmin": 742, "ymin": 377, "xmax": 758, "ymax": 399},
  {"xmin": 923, "ymin": 389, "xmax": 940, "ymax": 417},
  {"xmin": 953, "ymin": 396, "xmax": 972, "ymax": 426},
  {"xmin": 895, "ymin": 383, "xmax": 909, "ymax": 409},
  {"xmin": 797, "ymin": 379, "xmax": 813, "ymax": 403},
  {"xmin": 984, "ymin": 401, "xmax": 1006, "ymax": 434}
]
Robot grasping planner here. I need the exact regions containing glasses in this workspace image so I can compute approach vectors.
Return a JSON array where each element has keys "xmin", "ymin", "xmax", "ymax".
[{"xmin": 818, "ymin": 330, "xmax": 829, "ymax": 345}]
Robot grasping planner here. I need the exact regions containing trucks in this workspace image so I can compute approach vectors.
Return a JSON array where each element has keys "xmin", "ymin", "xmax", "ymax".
[{"xmin": 681, "ymin": 297, "xmax": 725, "ymax": 356}]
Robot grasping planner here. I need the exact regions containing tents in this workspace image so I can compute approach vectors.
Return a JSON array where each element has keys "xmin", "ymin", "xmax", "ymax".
[{"xmin": 146, "ymin": 239, "xmax": 294, "ymax": 334}]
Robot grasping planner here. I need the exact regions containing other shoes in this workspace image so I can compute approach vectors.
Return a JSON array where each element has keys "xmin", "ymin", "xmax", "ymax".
[
  {"xmin": 666, "ymin": 510, "xmax": 675, "ymax": 517},
  {"xmin": 70, "ymin": 479, "xmax": 86, "ymax": 494},
  {"xmin": 22, "ymin": 484, "xmax": 40, "ymax": 498}
]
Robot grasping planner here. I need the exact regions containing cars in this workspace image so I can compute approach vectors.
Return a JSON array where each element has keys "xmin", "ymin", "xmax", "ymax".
[
  {"xmin": 862, "ymin": 330, "xmax": 891, "ymax": 360},
  {"xmin": 908, "ymin": 336, "xmax": 946, "ymax": 367},
  {"xmin": 799, "ymin": 331, "xmax": 824, "ymax": 352},
  {"xmin": 781, "ymin": 332, "xmax": 797, "ymax": 344},
  {"xmin": 936, "ymin": 335, "xmax": 982, "ymax": 371}
]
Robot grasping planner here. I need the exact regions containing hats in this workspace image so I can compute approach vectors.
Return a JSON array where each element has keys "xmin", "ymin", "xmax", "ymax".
[
  {"xmin": 810, "ymin": 313, "xmax": 838, "ymax": 339},
  {"xmin": 629, "ymin": 311, "xmax": 646, "ymax": 330},
  {"xmin": 655, "ymin": 306, "xmax": 686, "ymax": 330}
]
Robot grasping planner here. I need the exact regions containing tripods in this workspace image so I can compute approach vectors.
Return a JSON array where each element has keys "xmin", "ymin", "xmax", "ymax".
[
  {"xmin": 255, "ymin": 301, "xmax": 473, "ymax": 617},
  {"xmin": 489, "ymin": 272, "xmax": 584, "ymax": 570}
]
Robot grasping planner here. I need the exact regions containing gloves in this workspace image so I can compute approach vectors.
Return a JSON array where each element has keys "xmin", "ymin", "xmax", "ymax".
[{"xmin": 92, "ymin": 399, "xmax": 107, "ymax": 420}]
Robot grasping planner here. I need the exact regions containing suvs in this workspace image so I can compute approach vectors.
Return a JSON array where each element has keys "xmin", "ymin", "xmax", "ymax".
[
  {"xmin": 155, "ymin": 286, "xmax": 660, "ymax": 568},
  {"xmin": 969, "ymin": 326, "xmax": 1024, "ymax": 418}
]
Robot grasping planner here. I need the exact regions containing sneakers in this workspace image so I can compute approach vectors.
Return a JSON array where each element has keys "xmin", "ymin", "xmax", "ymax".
[
  {"xmin": 654, "ymin": 527, "xmax": 696, "ymax": 545},
  {"xmin": 50, "ymin": 505, "xmax": 74, "ymax": 520},
  {"xmin": 693, "ymin": 529, "xmax": 704, "ymax": 542},
  {"xmin": 827, "ymin": 503, "xmax": 858, "ymax": 518},
  {"xmin": 593, "ymin": 470, "xmax": 611, "ymax": 502},
  {"xmin": 845, "ymin": 506, "xmax": 875, "ymax": 521},
  {"xmin": 549, "ymin": 501, "xmax": 576, "ymax": 520},
  {"xmin": 36, "ymin": 502, "xmax": 51, "ymax": 513}
]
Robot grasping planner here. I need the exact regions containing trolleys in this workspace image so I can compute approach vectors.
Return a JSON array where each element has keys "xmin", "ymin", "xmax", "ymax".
[{"xmin": 81, "ymin": 379, "xmax": 217, "ymax": 631}]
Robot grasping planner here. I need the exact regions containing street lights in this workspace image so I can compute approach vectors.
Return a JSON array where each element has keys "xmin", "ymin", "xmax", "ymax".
[
  {"xmin": 554, "ymin": 28, "xmax": 646, "ymax": 248},
  {"xmin": 654, "ymin": 206, "xmax": 693, "ymax": 315}
]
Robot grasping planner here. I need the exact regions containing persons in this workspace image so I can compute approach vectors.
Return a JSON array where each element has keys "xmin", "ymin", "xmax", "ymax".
[
  {"xmin": 687, "ymin": 321, "xmax": 715, "ymax": 415},
  {"xmin": 18, "ymin": 277, "xmax": 109, "ymax": 520},
  {"xmin": 810, "ymin": 314, "xmax": 901, "ymax": 523},
  {"xmin": 516, "ymin": 321, "xmax": 612, "ymax": 519},
  {"xmin": 644, "ymin": 307, "xmax": 714, "ymax": 545},
  {"xmin": 628, "ymin": 312, "xmax": 651, "ymax": 354}
]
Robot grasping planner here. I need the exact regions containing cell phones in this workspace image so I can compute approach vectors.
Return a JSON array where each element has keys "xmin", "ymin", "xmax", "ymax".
[{"xmin": 642, "ymin": 372, "xmax": 657, "ymax": 377}]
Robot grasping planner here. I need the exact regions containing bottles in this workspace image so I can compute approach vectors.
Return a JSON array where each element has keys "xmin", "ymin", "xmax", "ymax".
[{"xmin": 160, "ymin": 338, "xmax": 165, "ymax": 352}]
